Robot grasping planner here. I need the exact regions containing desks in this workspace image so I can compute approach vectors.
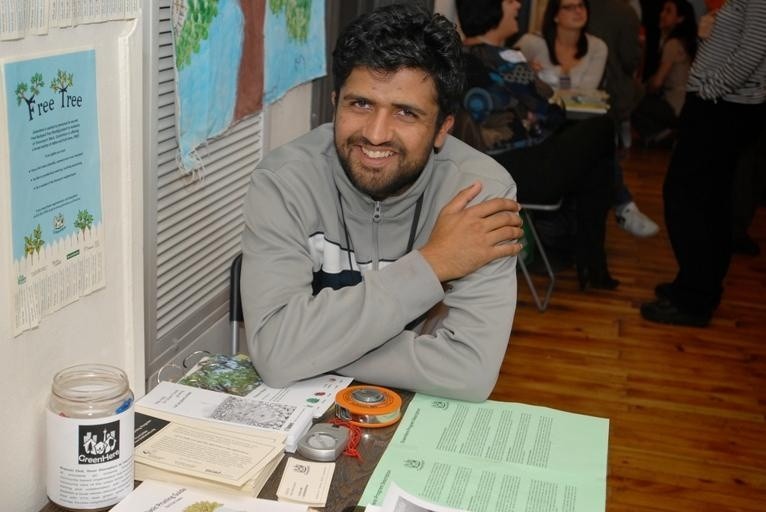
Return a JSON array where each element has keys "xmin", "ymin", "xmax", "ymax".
[{"xmin": 39, "ymin": 352, "xmax": 611, "ymax": 512}]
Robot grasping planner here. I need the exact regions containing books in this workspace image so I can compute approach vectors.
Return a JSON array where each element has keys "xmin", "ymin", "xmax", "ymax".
[{"xmin": 99, "ymin": 378, "xmax": 309, "ymax": 512}]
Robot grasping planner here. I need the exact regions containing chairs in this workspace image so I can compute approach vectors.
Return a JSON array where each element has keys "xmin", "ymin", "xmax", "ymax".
[
  {"xmin": 229, "ymin": 254, "xmax": 245, "ymax": 360},
  {"xmin": 459, "ymin": 116, "xmax": 567, "ymax": 312}
]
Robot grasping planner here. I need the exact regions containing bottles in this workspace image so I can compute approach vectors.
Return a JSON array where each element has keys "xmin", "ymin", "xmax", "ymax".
[{"xmin": 44, "ymin": 364, "xmax": 136, "ymax": 509}]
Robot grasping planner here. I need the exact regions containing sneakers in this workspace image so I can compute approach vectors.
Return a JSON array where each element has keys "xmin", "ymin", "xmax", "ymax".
[
  {"xmin": 576, "ymin": 273, "xmax": 726, "ymax": 329},
  {"xmin": 614, "ymin": 201, "xmax": 661, "ymax": 240}
]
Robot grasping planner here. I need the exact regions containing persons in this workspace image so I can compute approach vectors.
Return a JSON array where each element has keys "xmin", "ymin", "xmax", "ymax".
[
  {"xmin": 641, "ymin": 0, "xmax": 766, "ymax": 326},
  {"xmin": 236, "ymin": 0, "xmax": 525, "ymax": 403},
  {"xmin": 448, "ymin": 0, "xmax": 663, "ymax": 296}
]
